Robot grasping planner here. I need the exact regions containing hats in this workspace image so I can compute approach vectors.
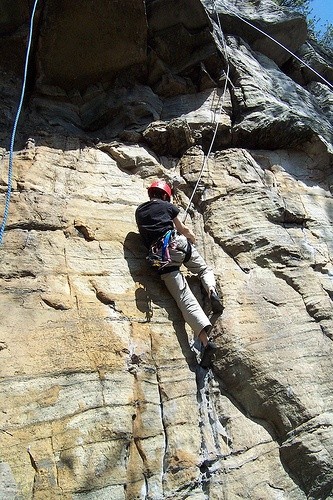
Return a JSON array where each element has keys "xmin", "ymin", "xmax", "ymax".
[{"xmin": 148, "ymin": 181, "xmax": 172, "ymax": 203}]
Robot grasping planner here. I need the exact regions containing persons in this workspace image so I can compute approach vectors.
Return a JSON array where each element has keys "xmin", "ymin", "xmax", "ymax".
[{"xmin": 136, "ymin": 182, "xmax": 225, "ymax": 368}]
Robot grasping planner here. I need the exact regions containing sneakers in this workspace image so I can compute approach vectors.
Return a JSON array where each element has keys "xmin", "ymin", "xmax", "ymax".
[
  {"xmin": 209, "ymin": 293, "xmax": 225, "ymax": 313},
  {"xmin": 201, "ymin": 341, "xmax": 218, "ymax": 368}
]
